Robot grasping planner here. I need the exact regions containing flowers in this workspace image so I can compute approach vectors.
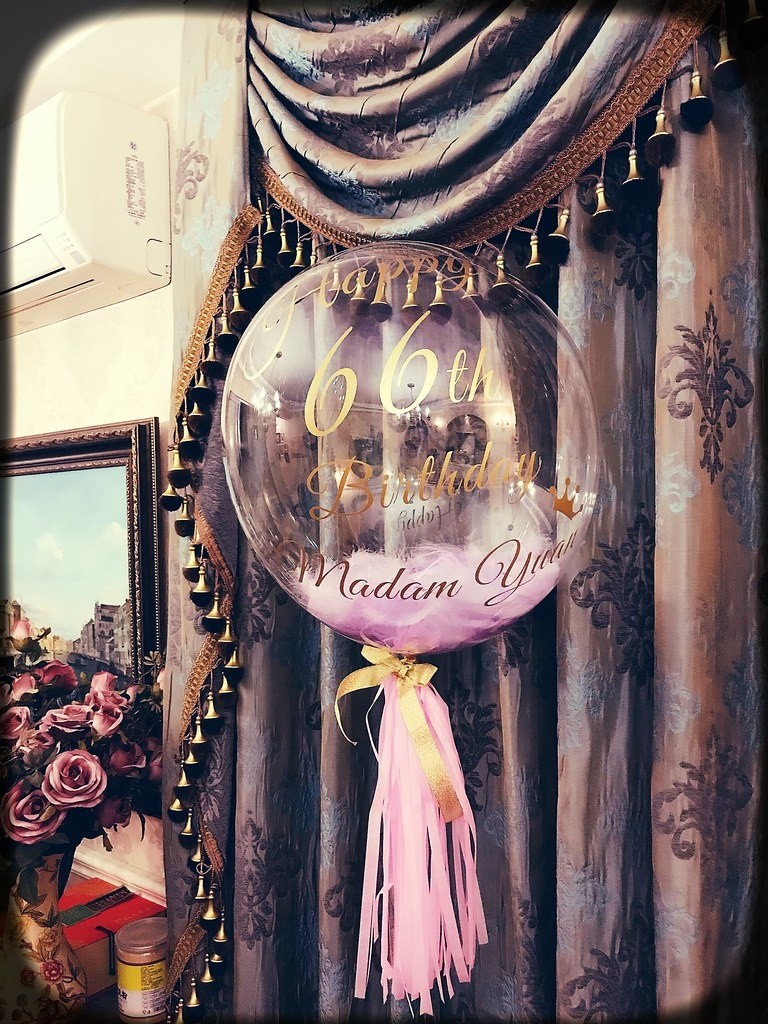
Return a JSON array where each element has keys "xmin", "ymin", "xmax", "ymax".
[{"xmin": 0, "ymin": 601, "xmax": 167, "ymax": 851}]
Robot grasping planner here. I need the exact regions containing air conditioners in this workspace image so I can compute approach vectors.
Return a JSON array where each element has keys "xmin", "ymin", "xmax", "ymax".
[{"xmin": 0, "ymin": 86, "xmax": 174, "ymax": 343}]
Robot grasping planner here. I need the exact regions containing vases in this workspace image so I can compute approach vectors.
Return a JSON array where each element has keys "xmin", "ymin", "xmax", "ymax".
[{"xmin": 0, "ymin": 851, "xmax": 88, "ymax": 1024}]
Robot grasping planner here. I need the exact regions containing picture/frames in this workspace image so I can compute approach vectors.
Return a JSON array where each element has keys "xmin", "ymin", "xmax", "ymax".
[{"xmin": 1, "ymin": 418, "xmax": 164, "ymax": 821}]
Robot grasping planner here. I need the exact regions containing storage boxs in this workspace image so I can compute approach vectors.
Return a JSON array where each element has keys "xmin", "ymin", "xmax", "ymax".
[{"xmin": 57, "ymin": 877, "xmax": 169, "ymax": 1004}]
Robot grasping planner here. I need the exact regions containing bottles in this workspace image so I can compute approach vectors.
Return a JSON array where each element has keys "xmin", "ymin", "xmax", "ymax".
[{"xmin": 114, "ymin": 916, "xmax": 168, "ymax": 1023}]
